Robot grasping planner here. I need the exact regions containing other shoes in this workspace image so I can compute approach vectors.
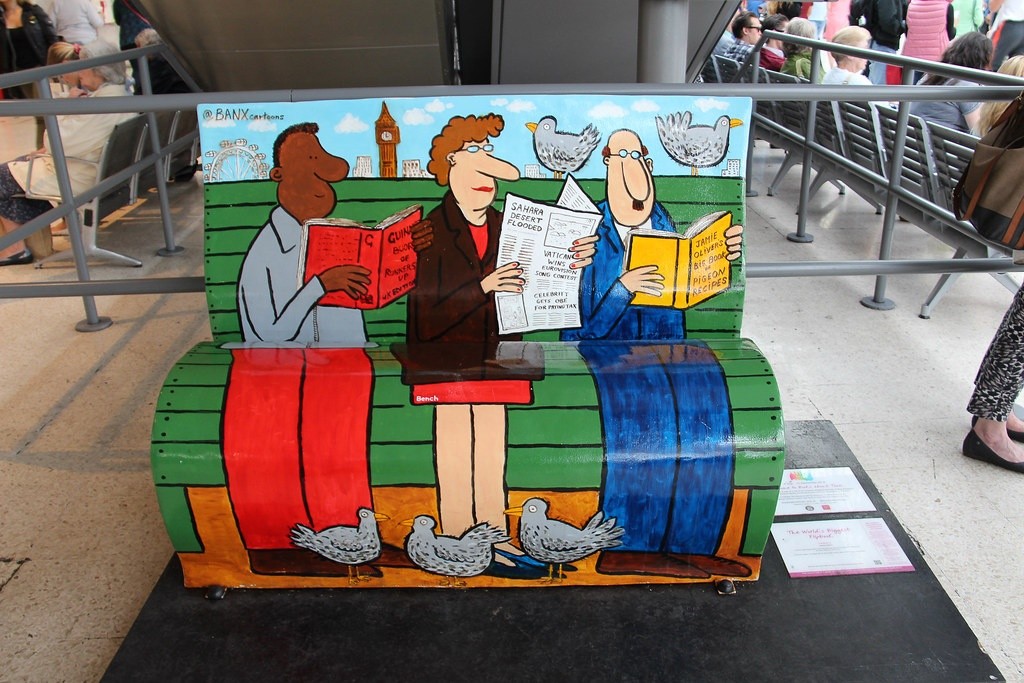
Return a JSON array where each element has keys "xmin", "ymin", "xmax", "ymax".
[
  {"xmin": 962, "ymin": 429, "xmax": 1024, "ymax": 473},
  {"xmin": 971, "ymin": 415, "xmax": 1024, "ymax": 444}
]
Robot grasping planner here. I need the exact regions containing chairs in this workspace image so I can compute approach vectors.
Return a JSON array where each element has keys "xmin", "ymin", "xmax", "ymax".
[
  {"xmin": 12, "ymin": 111, "xmax": 201, "ymax": 269},
  {"xmin": 702, "ymin": 54, "xmax": 1024, "ymax": 320}
]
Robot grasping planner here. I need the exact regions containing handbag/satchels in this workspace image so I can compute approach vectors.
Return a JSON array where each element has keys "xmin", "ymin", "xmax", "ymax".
[{"xmin": 950, "ymin": 88, "xmax": 1023, "ymax": 251}]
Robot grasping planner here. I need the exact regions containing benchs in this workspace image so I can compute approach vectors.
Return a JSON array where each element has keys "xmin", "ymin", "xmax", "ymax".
[{"xmin": 149, "ymin": 94, "xmax": 784, "ymax": 599}]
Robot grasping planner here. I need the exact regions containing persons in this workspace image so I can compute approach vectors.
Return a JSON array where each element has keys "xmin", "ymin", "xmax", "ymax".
[
  {"xmin": 0, "ymin": 0, "xmax": 201, "ymax": 268},
  {"xmin": 711, "ymin": 0, "xmax": 1024, "ymax": 474}
]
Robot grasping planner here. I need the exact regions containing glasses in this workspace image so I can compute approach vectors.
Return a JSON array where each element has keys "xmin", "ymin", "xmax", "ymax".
[{"xmin": 746, "ymin": 25, "xmax": 762, "ymax": 30}]
